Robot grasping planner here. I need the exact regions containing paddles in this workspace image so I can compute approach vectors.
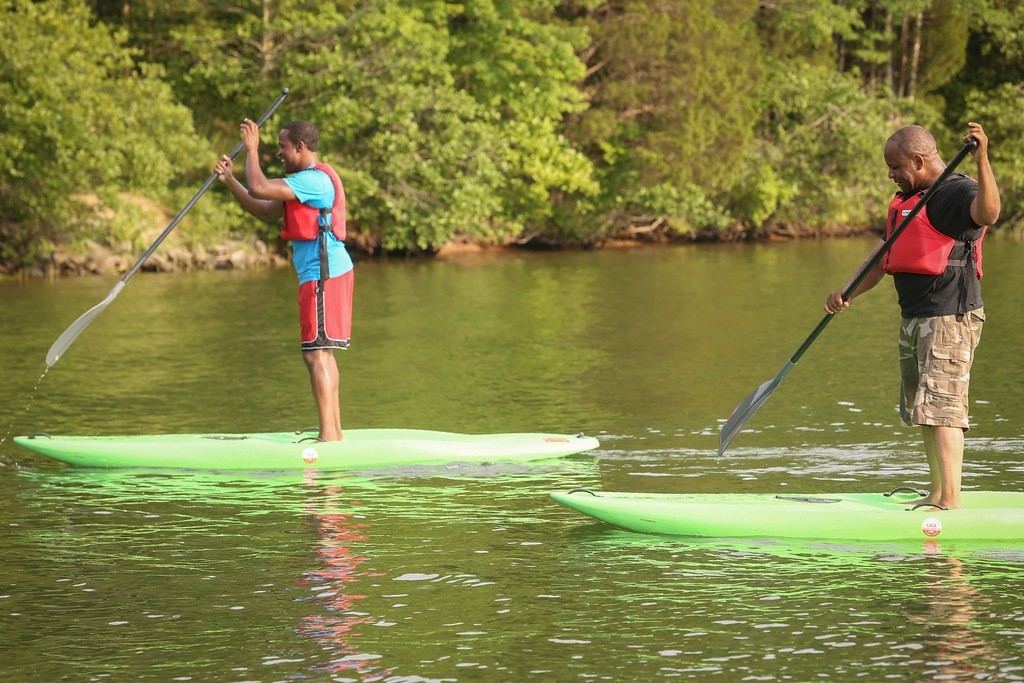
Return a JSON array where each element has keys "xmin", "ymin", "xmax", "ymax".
[
  {"xmin": 43, "ymin": 84, "xmax": 293, "ymax": 369},
  {"xmin": 716, "ymin": 138, "xmax": 980, "ymax": 458}
]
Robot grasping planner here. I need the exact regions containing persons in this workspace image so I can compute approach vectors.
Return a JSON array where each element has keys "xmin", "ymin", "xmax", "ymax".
[
  {"xmin": 825, "ymin": 121, "xmax": 1001, "ymax": 512},
  {"xmin": 213, "ymin": 118, "xmax": 354, "ymax": 443}
]
